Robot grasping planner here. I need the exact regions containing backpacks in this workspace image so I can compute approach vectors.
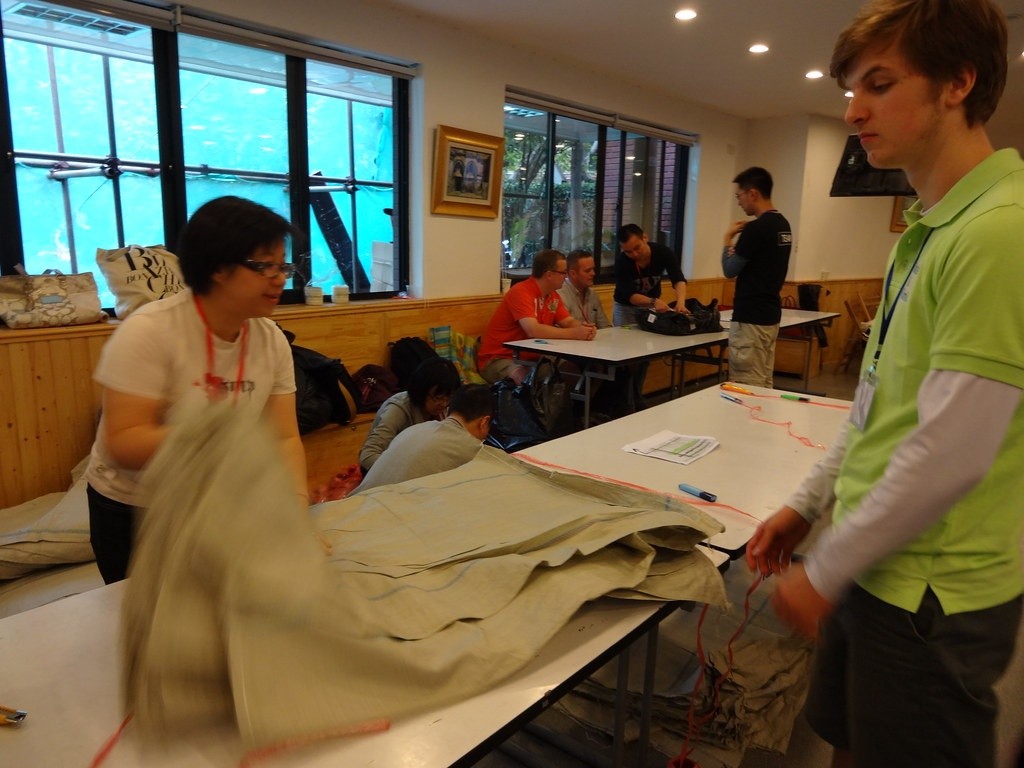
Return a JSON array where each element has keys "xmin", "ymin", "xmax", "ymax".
[
  {"xmin": 484, "ymin": 357, "xmax": 584, "ymax": 454},
  {"xmin": 276, "ymin": 322, "xmax": 461, "ymax": 434},
  {"xmin": 635, "ymin": 298, "xmax": 724, "ymax": 336}
]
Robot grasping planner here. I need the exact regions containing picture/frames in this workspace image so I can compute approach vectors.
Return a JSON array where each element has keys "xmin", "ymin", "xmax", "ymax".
[
  {"xmin": 890, "ymin": 196, "xmax": 909, "ymax": 233},
  {"xmin": 431, "ymin": 125, "xmax": 506, "ymax": 218}
]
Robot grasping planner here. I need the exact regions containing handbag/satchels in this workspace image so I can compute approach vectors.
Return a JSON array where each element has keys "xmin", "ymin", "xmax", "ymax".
[
  {"xmin": 96, "ymin": 245, "xmax": 190, "ymax": 321},
  {"xmin": 0, "ymin": 268, "xmax": 108, "ymax": 327}
]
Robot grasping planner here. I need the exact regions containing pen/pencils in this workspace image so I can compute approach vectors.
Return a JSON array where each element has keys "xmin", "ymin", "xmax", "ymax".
[{"xmin": 720, "ymin": 393, "xmax": 742, "ymax": 404}]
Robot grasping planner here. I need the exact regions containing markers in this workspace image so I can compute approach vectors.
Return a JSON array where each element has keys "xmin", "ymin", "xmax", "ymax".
[
  {"xmin": 721, "ymin": 383, "xmax": 754, "ymax": 395},
  {"xmin": 678, "ymin": 483, "xmax": 718, "ymax": 502},
  {"xmin": 781, "ymin": 394, "xmax": 810, "ymax": 402}
]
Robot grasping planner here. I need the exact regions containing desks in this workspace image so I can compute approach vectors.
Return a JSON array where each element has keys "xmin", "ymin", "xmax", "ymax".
[{"xmin": 0, "ymin": 299, "xmax": 853, "ymax": 767}]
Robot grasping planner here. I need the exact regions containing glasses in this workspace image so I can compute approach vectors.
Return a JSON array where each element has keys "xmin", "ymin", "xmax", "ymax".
[
  {"xmin": 552, "ymin": 269, "xmax": 567, "ymax": 276},
  {"xmin": 239, "ymin": 259, "xmax": 296, "ymax": 279},
  {"xmin": 430, "ymin": 393, "xmax": 449, "ymax": 403},
  {"xmin": 735, "ymin": 190, "xmax": 750, "ymax": 199}
]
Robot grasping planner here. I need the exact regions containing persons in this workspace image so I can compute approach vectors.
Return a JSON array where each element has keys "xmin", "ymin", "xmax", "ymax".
[
  {"xmin": 746, "ymin": 0, "xmax": 1024, "ymax": 768},
  {"xmin": 555, "ymin": 250, "xmax": 612, "ymax": 328},
  {"xmin": 722, "ymin": 167, "xmax": 792, "ymax": 389},
  {"xmin": 612, "ymin": 224, "xmax": 691, "ymax": 411},
  {"xmin": 358, "ymin": 357, "xmax": 461, "ymax": 481},
  {"xmin": 87, "ymin": 196, "xmax": 308, "ymax": 586},
  {"xmin": 477, "ymin": 249, "xmax": 597, "ymax": 386},
  {"xmin": 345, "ymin": 383, "xmax": 496, "ymax": 498}
]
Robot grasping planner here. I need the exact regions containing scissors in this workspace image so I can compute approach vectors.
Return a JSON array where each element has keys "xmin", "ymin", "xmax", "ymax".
[{"xmin": 534, "ymin": 340, "xmax": 553, "ymax": 345}]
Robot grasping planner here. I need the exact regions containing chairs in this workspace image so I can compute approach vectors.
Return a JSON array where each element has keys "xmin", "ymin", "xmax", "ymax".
[{"xmin": 835, "ymin": 292, "xmax": 872, "ymax": 375}]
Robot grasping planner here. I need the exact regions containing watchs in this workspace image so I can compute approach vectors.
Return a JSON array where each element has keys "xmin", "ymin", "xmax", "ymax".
[{"xmin": 651, "ymin": 297, "xmax": 657, "ymax": 308}]
